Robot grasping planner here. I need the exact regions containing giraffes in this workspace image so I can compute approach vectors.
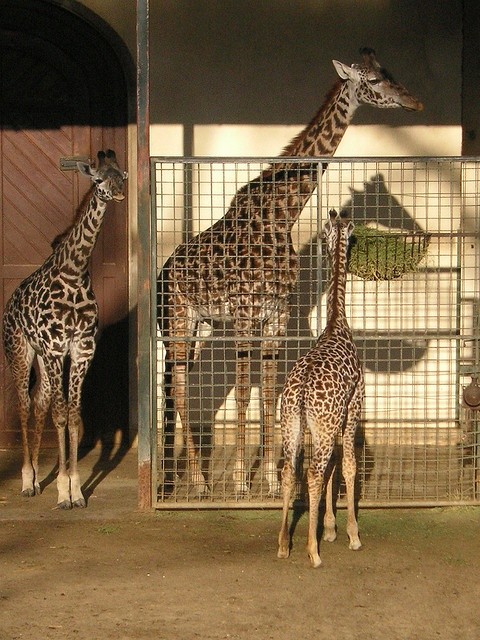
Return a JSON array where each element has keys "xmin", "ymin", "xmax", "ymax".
[
  {"xmin": 157, "ymin": 46, "xmax": 424, "ymax": 498},
  {"xmin": 1, "ymin": 149, "xmax": 128, "ymax": 508},
  {"xmin": 277, "ymin": 208, "xmax": 365, "ymax": 571}
]
